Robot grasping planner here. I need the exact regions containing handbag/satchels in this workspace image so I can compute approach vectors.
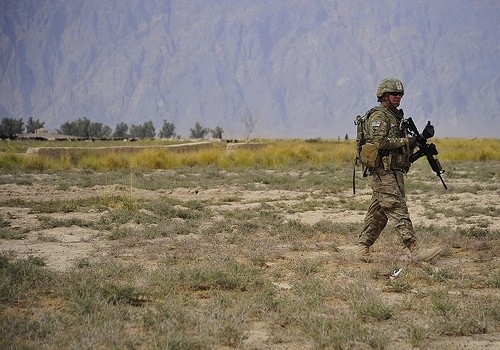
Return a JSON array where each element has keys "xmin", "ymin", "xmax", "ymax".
[{"xmin": 359, "ymin": 141, "xmax": 380, "ymax": 169}]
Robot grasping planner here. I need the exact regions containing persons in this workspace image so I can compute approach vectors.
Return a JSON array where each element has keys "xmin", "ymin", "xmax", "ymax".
[{"xmin": 353, "ymin": 78, "xmax": 442, "ymax": 263}]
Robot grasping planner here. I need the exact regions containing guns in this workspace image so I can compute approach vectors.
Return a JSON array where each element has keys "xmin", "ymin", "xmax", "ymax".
[{"xmin": 401, "ymin": 117, "xmax": 448, "ymax": 190}]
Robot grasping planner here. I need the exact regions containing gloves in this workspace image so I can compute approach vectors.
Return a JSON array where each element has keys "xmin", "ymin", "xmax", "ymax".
[{"xmin": 422, "ymin": 120, "xmax": 434, "ymax": 138}]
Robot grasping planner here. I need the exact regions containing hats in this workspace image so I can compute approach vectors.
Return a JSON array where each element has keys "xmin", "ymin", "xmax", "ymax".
[{"xmin": 377, "ymin": 78, "xmax": 404, "ymax": 96}]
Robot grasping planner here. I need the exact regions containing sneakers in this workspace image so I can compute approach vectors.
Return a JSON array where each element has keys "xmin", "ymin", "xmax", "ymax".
[
  {"xmin": 356, "ymin": 245, "xmax": 379, "ymax": 263},
  {"xmin": 411, "ymin": 246, "xmax": 442, "ymax": 261}
]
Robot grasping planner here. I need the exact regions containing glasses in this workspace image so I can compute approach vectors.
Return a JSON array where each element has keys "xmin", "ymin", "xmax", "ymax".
[{"xmin": 390, "ymin": 93, "xmax": 401, "ymax": 96}]
